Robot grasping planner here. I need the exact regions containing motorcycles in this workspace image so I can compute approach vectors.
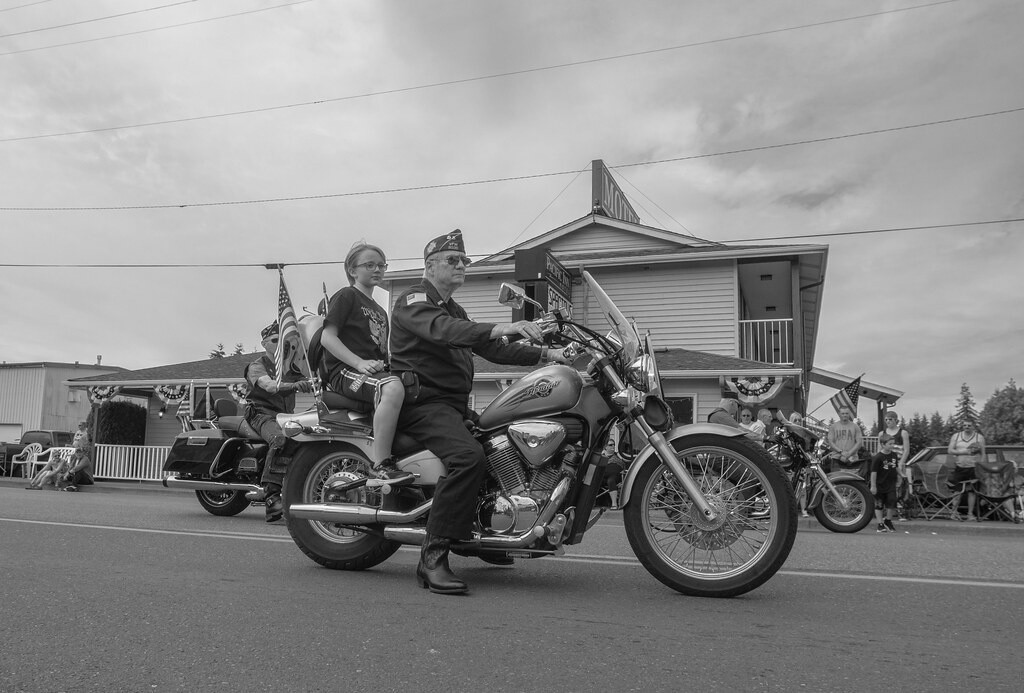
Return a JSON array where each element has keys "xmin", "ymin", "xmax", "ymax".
[
  {"xmin": 162, "ymin": 397, "xmax": 313, "ymax": 516},
  {"xmin": 276, "ymin": 269, "xmax": 801, "ymax": 598},
  {"xmin": 766, "ymin": 410, "xmax": 875, "ymax": 533}
]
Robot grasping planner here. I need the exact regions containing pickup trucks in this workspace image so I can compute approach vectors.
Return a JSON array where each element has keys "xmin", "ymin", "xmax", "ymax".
[{"xmin": 0, "ymin": 430, "xmax": 83, "ymax": 478}]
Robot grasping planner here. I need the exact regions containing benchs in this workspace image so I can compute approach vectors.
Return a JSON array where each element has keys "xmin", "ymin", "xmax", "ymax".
[{"xmin": 30, "ymin": 447, "xmax": 82, "ymax": 479}]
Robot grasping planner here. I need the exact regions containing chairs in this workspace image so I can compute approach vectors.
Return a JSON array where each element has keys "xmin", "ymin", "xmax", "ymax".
[
  {"xmin": 906, "ymin": 461, "xmax": 970, "ymax": 522},
  {"xmin": 10, "ymin": 443, "xmax": 42, "ymax": 480},
  {"xmin": 970, "ymin": 461, "xmax": 1019, "ymax": 523}
]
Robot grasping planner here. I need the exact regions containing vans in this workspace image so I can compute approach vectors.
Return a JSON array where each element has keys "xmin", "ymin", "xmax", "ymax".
[{"xmin": 905, "ymin": 445, "xmax": 1024, "ymax": 492}]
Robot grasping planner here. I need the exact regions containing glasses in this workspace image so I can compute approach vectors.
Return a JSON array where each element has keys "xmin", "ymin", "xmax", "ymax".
[
  {"xmin": 266, "ymin": 338, "xmax": 279, "ymax": 343},
  {"xmin": 436, "ymin": 257, "xmax": 472, "ymax": 267},
  {"xmin": 742, "ymin": 415, "xmax": 750, "ymax": 418},
  {"xmin": 355, "ymin": 262, "xmax": 388, "ymax": 271},
  {"xmin": 963, "ymin": 425, "xmax": 971, "ymax": 427}
]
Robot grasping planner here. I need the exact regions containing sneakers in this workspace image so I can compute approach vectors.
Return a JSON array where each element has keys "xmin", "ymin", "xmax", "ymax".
[{"xmin": 365, "ymin": 456, "xmax": 416, "ymax": 487}]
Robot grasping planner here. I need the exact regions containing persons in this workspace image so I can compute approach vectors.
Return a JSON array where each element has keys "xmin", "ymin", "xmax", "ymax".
[
  {"xmin": 307, "ymin": 229, "xmax": 579, "ymax": 594},
  {"xmin": 706, "ymin": 391, "xmax": 986, "ymax": 532},
  {"xmin": 244, "ymin": 320, "xmax": 311, "ymax": 522},
  {"xmin": 72, "ymin": 421, "xmax": 91, "ymax": 448},
  {"xmin": 25, "ymin": 448, "xmax": 94, "ymax": 492}
]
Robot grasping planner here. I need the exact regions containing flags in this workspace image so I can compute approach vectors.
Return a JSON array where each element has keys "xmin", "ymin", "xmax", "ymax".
[
  {"xmin": 273, "ymin": 276, "xmax": 300, "ymax": 393},
  {"xmin": 176, "ymin": 386, "xmax": 193, "ymax": 432}
]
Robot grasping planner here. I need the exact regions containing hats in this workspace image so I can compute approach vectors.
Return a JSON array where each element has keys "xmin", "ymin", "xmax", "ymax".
[
  {"xmin": 75, "ymin": 447, "xmax": 83, "ymax": 452},
  {"xmin": 880, "ymin": 434, "xmax": 893, "ymax": 443},
  {"xmin": 721, "ymin": 391, "xmax": 738, "ymax": 399},
  {"xmin": 261, "ymin": 323, "xmax": 280, "ymax": 340},
  {"xmin": 424, "ymin": 229, "xmax": 465, "ymax": 262}
]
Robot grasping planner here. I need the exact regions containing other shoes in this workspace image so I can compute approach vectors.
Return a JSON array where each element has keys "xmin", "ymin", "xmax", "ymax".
[
  {"xmin": 883, "ymin": 518, "xmax": 895, "ymax": 533},
  {"xmin": 951, "ymin": 512, "xmax": 961, "ymax": 521},
  {"xmin": 967, "ymin": 514, "xmax": 976, "ymax": 522},
  {"xmin": 877, "ymin": 524, "xmax": 886, "ymax": 533},
  {"xmin": 62, "ymin": 486, "xmax": 78, "ymax": 492},
  {"xmin": 25, "ymin": 485, "xmax": 42, "ymax": 490}
]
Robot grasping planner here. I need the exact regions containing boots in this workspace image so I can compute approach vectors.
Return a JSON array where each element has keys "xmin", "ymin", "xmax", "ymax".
[
  {"xmin": 264, "ymin": 483, "xmax": 283, "ymax": 522},
  {"xmin": 416, "ymin": 532, "xmax": 469, "ymax": 593},
  {"xmin": 479, "ymin": 550, "xmax": 515, "ymax": 564}
]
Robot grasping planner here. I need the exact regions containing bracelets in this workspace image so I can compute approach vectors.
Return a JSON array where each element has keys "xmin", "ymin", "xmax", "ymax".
[{"xmin": 542, "ymin": 349, "xmax": 548, "ymax": 363}]
